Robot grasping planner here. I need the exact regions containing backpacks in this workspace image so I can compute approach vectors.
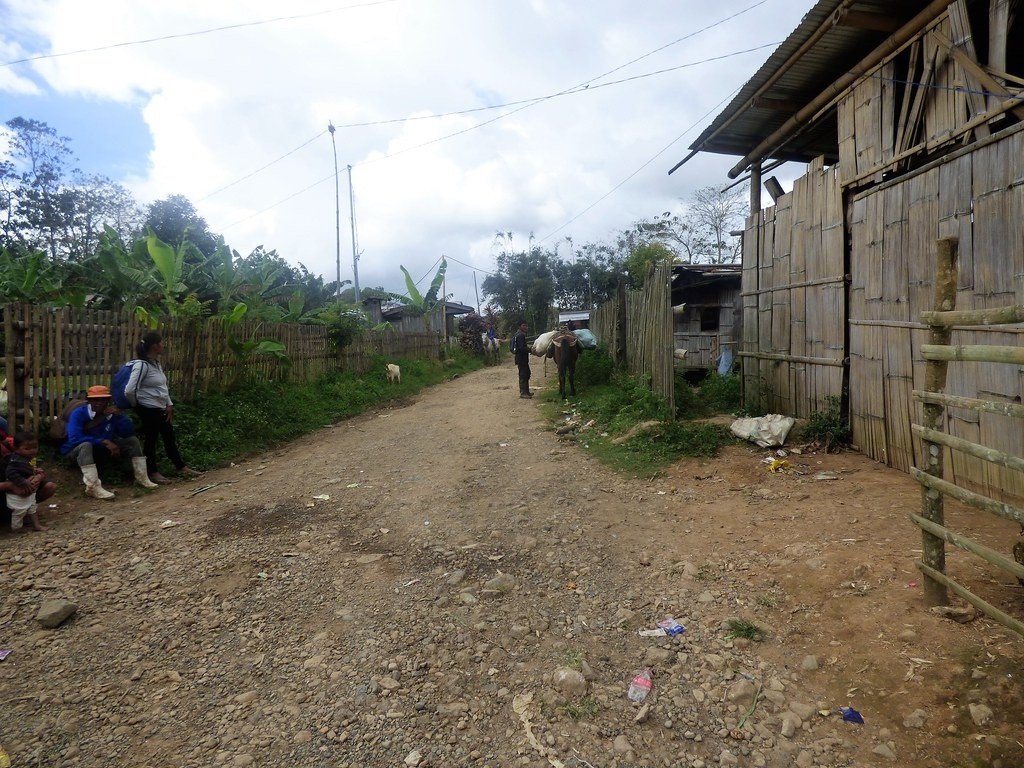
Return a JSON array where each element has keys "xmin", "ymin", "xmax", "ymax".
[
  {"xmin": 510, "ymin": 332, "xmax": 525, "ymax": 354},
  {"xmin": 112, "ymin": 359, "xmax": 146, "ymax": 409}
]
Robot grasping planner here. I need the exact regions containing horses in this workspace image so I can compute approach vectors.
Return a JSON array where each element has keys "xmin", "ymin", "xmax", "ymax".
[
  {"xmin": 481, "ymin": 331, "xmax": 501, "ymax": 367},
  {"xmin": 552, "ymin": 320, "xmax": 581, "ymax": 402}
]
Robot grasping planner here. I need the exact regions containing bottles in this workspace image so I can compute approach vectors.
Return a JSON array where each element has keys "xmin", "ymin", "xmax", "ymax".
[{"xmin": 628, "ymin": 668, "xmax": 652, "ymax": 702}]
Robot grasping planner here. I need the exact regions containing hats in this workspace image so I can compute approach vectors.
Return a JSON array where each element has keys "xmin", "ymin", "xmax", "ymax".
[
  {"xmin": 0, "ymin": 417, "xmax": 8, "ymax": 441},
  {"xmin": 85, "ymin": 385, "xmax": 113, "ymax": 398}
]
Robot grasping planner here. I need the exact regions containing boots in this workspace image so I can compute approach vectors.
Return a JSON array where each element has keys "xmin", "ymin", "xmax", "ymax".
[
  {"xmin": 80, "ymin": 464, "xmax": 117, "ymax": 500},
  {"xmin": 131, "ymin": 455, "xmax": 159, "ymax": 490}
]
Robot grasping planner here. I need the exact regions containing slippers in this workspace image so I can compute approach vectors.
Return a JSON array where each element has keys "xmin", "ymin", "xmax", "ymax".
[
  {"xmin": 175, "ymin": 471, "xmax": 202, "ymax": 478},
  {"xmin": 151, "ymin": 475, "xmax": 171, "ymax": 485}
]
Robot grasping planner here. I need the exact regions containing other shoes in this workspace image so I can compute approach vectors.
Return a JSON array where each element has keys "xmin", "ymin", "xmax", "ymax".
[
  {"xmin": 527, "ymin": 392, "xmax": 534, "ymax": 396},
  {"xmin": 520, "ymin": 394, "xmax": 531, "ymax": 399}
]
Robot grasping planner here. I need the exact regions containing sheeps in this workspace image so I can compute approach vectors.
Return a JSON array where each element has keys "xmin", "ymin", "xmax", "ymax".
[
  {"xmin": 0, "ymin": 367, "xmax": 88, "ymax": 423},
  {"xmin": 383, "ymin": 360, "xmax": 401, "ymax": 384}
]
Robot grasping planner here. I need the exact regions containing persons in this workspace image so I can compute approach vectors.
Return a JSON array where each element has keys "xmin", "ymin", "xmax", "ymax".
[
  {"xmin": 0, "ymin": 416, "xmax": 57, "ymax": 528},
  {"xmin": 62, "ymin": 385, "xmax": 160, "ymax": 500},
  {"xmin": 485, "ymin": 322, "xmax": 497, "ymax": 355},
  {"xmin": 4, "ymin": 431, "xmax": 50, "ymax": 534},
  {"xmin": 513, "ymin": 320, "xmax": 535, "ymax": 399},
  {"xmin": 125, "ymin": 330, "xmax": 208, "ymax": 484}
]
